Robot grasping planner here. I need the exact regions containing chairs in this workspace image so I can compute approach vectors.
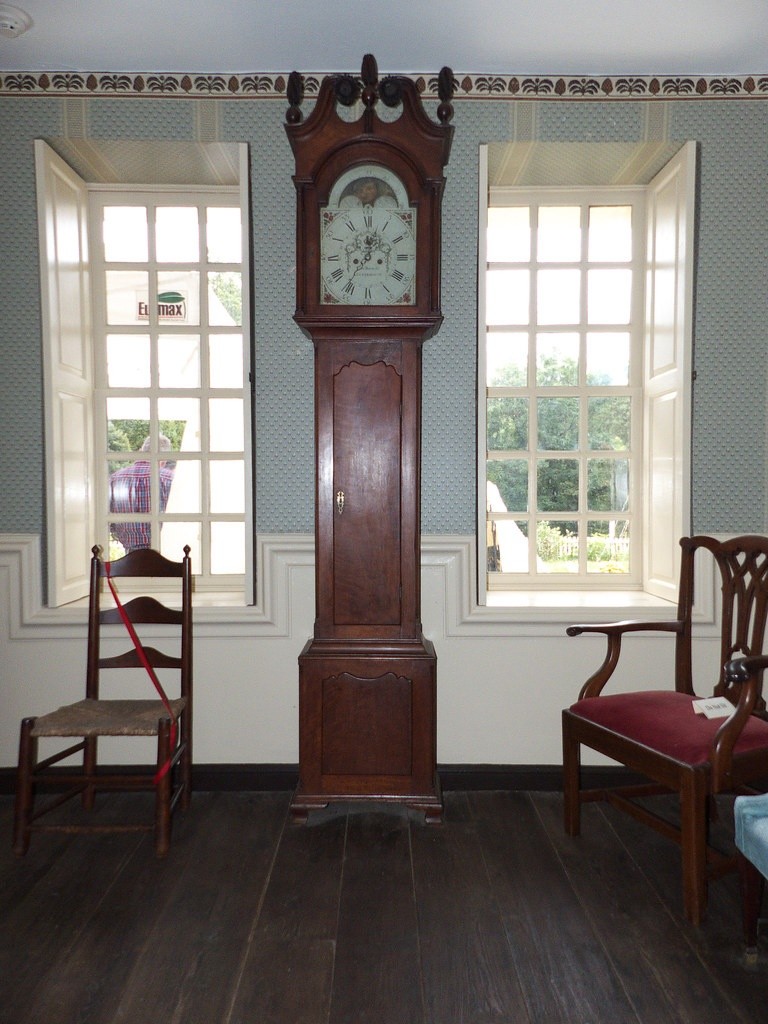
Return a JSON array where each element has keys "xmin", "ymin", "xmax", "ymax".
[
  {"xmin": 17, "ymin": 545, "xmax": 194, "ymax": 857},
  {"xmin": 556, "ymin": 533, "xmax": 768, "ymax": 920}
]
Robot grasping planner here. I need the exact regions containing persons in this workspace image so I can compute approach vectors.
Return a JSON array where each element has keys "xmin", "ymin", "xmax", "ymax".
[{"xmin": 107, "ymin": 435, "xmax": 173, "ymax": 556}]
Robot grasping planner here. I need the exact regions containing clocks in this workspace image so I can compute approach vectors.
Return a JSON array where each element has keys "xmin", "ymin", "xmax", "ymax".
[{"xmin": 280, "ymin": 51, "xmax": 459, "ymax": 830}]
